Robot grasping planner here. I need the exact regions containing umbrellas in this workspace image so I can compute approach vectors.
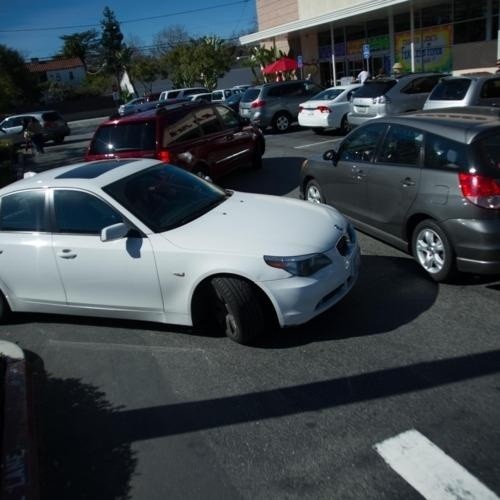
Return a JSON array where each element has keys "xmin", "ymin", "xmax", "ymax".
[{"xmin": 263, "ymin": 56, "xmax": 298, "ymax": 74}]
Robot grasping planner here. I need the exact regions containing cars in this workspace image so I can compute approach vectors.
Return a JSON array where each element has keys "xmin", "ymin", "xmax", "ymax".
[
  {"xmin": 1, "ymin": 109, "xmax": 70, "ymax": 147},
  {"xmin": 1, "ymin": 159, "xmax": 360, "ymax": 343},
  {"xmin": 296, "ymin": 114, "xmax": 500, "ymax": 284}
]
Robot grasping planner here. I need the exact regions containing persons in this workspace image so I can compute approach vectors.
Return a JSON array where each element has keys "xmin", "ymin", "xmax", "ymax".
[
  {"xmin": 24, "ymin": 117, "xmax": 45, "ymax": 154},
  {"xmin": 305, "ymin": 74, "xmax": 314, "ymax": 81},
  {"xmin": 112, "ymin": 85, "xmax": 121, "ymax": 107},
  {"xmin": 392, "ymin": 62, "xmax": 404, "ymax": 78},
  {"xmin": 496, "ymin": 60, "xmax": 500, "ymax": 74},
  {"xmin": 357, "ymin": 68, "xmax": 370, "ymax": 84},
  {"xmin": 278, "ymin": 72, "xmax": 282, "ymax": 81}
]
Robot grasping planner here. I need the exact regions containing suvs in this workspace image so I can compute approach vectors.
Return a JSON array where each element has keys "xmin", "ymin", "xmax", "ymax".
[{"xmin": 84, "ymin": 101, "xmax": 267, "ymax": 187}]
